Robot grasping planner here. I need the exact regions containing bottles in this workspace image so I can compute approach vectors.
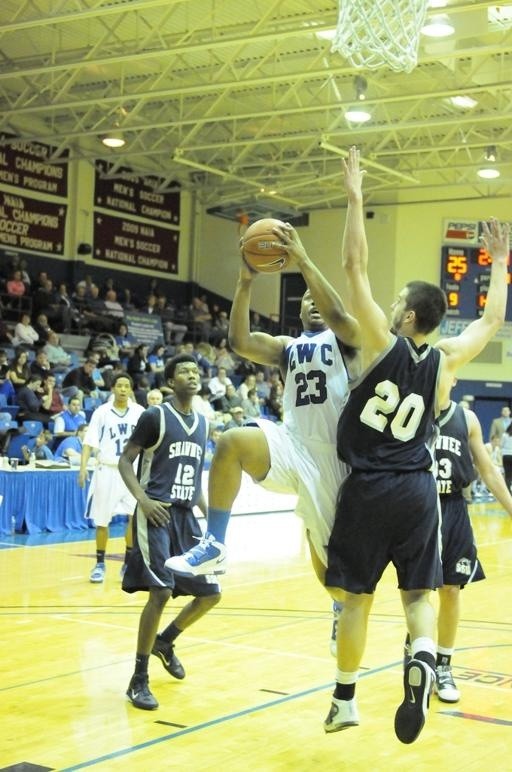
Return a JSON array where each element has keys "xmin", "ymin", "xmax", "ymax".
[{"xmin": 30, "ymin": 452, "xmax": 35, "ymax": 467}]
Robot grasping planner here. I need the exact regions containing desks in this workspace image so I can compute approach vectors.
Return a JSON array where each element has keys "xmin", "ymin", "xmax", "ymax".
[{"xmin": 0, "ymin": 459, "xmax": 128, "ymax": 537}]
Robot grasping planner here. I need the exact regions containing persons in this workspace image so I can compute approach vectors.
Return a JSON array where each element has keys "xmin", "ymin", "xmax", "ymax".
[
  {"xmin": 323, "ymin": 145, "xmax": 509, "ymax": 744},
  {"xmin": 164, "ymin": 218, "xmax": 364, "ymax": 657},
  {"xmin": 165, "ymin": 280, "xmax": 262, "ymax": 368},
  {"xmin": 207, "ymin": 366, "xmax": 285, "ymax": 427},
  {"xmin": 104, "ymin": 271, "xmax": 164, "ymax": 408},
  {"xmin": 120, "ymin": 357, "xmax": 209, "ymax": 710},
  {"xmin": 1, "ymin": 265, "xmax": 103, "ymax": 385},
  {"xmin": 80, "ymin": 377, "xmax": 147, "ymax": 585},
  {"xmin": 458, "ymin": 399, "xmax": 511, "ymax": 505},
  {"xmin": 428, "ymin": 347, "xmax": 511, "ymax": 705},
  {"xmin": 0, "ymin": 385, "xmax": 81, "ymax": 466}
]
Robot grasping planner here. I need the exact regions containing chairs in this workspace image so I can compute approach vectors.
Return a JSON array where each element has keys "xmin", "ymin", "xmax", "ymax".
[{"xmin": 1, "ymin": 336, "xmax": 282, "ymax": 448}]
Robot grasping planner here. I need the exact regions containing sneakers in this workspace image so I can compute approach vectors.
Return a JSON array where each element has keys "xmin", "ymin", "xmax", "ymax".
[
  {"xmin": 89, "ymin": 562, "xmax": 105, "ymax": 582},
  {"xmin": 163, "ymin": 530, "xmax": 228, "ymax": 577},
  {"xmin": 323, "ymin": 695, "xmax": 360, "ymax": 733},
  {"xmin": 126, "ymin": 673, "xmax": 159, "ymax": 710},
  {"xmin": 401, "ymin": 632, "xmax": 414, "ymax": 670},
  {"xmin": 394, "ymin": 658, "xmax": 438, "ymax": 745},
  {"xmin": 329, "ymin": 600, "xmax": 342, "ymax": 658},
  {"xmin": 150, "ymin": 632, "xmax": 185, "ymax": 679},
  {"xmin": 433, "ymin": 665, "xmax": 461, "ymax": 703}
]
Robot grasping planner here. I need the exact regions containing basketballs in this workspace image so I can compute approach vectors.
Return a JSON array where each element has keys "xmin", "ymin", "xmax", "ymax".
[{"xmin": 242, "ymin": 217, "xmax": 290, "ymax": 273}]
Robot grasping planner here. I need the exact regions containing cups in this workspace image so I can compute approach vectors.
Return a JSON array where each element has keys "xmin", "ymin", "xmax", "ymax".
[{"xmin": 11, "ymin": 457, "xmax": 18, "ymax": 470}]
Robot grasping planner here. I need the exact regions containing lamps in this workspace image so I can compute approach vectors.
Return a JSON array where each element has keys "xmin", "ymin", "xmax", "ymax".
[
  {"xmin": 489, "ymin": 5, "xmax": 509, "ymax": 26},
  {"xmin": 476, "ymin": 167, "xmax": 498, "ymax": 182},
  {"xmin": 483, "ymin": 142, "xmax": 497, "ymax": 163},
  {"xmin": 448, "ymin": 94, "xmax": 477, "ymax": 114},
  {"xmin": 421, "ymin": 12, "xmax": 451, "ymax": 38},
  {"xmin": 102, "ymin": 64, "xmax": 126, "ymax": 147},
  {"xmin": 344, "ymin": 71, "xmax": 371, "ymax": 127}
]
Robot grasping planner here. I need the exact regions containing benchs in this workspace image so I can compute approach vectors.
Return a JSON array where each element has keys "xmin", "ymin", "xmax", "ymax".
[{"xmin": 0, "ymin": 276, "xmax": 270, "ymax": 346}]
